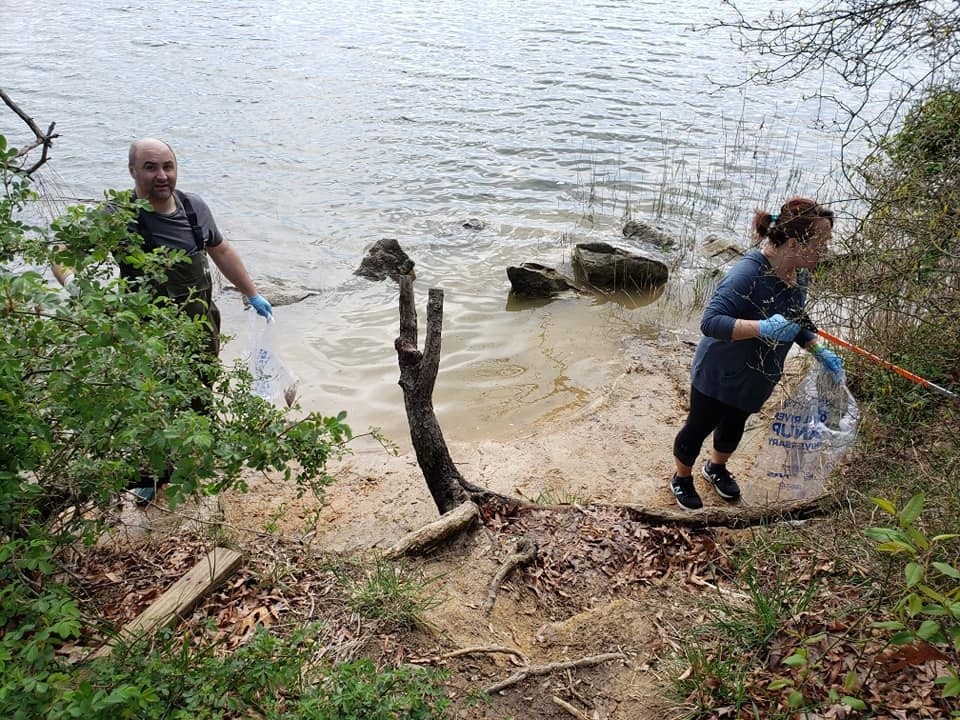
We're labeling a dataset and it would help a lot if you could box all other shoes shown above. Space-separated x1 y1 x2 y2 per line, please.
135 487 156 506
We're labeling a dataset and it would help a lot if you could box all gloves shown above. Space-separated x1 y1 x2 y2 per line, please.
64 274 93 299
760 314 800 342
248 293 272 319
816 347 841 375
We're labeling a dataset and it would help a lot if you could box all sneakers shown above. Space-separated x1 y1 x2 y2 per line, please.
702 459 740 498
670 472 704 515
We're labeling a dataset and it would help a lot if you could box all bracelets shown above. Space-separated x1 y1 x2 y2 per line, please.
808 342 822 352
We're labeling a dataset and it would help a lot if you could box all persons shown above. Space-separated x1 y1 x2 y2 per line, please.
669 199 842 509
52 138 272 421
